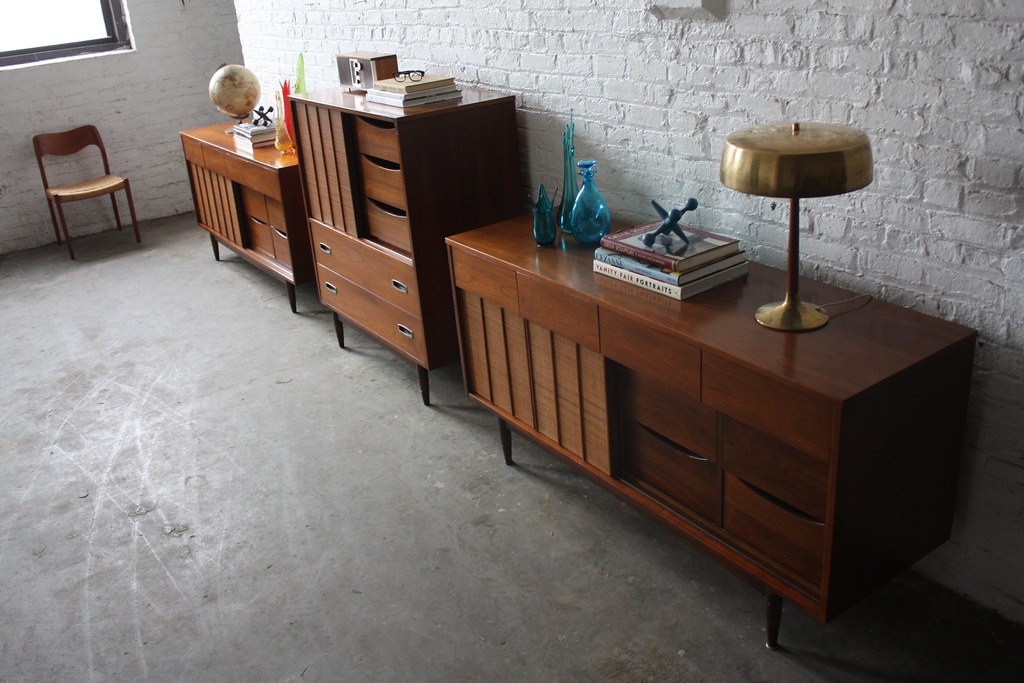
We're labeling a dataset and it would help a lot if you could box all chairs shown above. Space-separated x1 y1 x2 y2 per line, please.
32 125 141 260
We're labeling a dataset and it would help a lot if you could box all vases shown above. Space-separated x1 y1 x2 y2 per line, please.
294 53 306 95
272 90 296 156
558 108 580 233
279 78 297 149
528 184 560 245
568 159 611 244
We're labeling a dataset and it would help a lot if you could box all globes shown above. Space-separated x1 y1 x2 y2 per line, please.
209 63 261 134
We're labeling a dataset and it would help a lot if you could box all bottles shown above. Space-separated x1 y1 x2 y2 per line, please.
571 159 612 246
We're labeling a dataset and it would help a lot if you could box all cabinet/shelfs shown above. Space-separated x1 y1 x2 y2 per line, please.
444 209 979 649
179 119 316 313
287 82 525 407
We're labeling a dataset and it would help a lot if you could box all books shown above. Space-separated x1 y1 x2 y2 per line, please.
601 221 741 270
592 245 748 300
365 73 465 107
233 117 277 149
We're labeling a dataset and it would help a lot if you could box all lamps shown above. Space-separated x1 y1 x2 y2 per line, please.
720 120 874 332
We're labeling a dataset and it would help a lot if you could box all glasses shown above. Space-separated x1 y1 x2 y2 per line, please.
392 70 425 83
